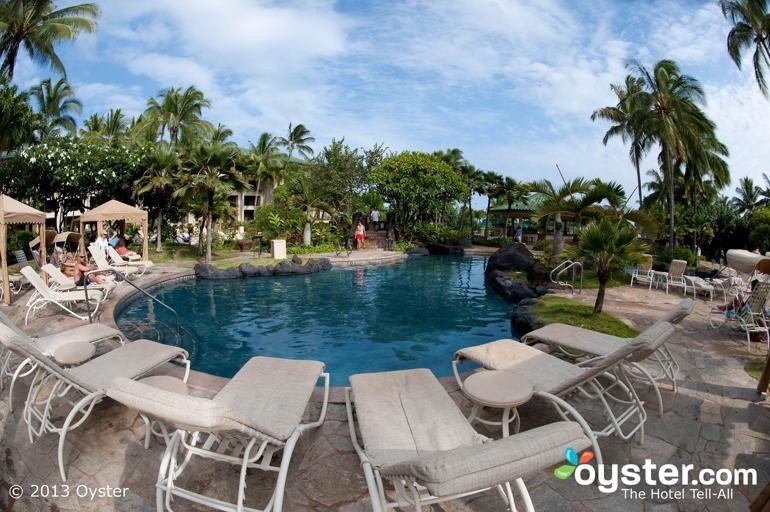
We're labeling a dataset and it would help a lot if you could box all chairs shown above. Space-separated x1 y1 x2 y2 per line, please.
628 253 770 352
0 298 697 512
1 242 155 328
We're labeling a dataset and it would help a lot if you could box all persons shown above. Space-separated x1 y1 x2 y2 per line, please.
715 278 761 319
25 221 129 291
354 219 367 249
494 222 522 245
351 267 364 297
371 207 380 233
133 222 208 244
